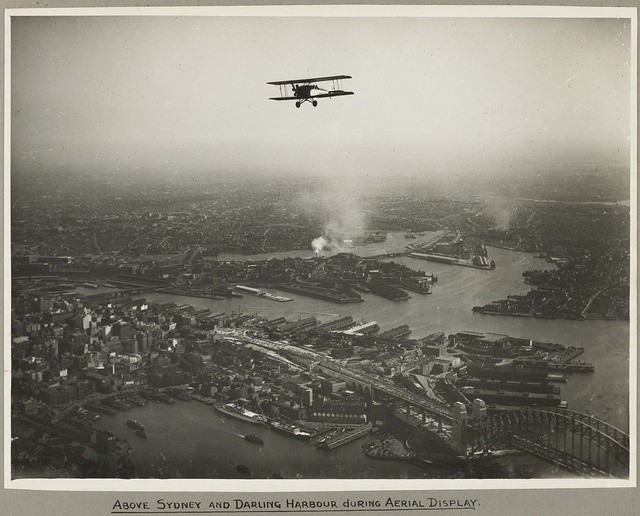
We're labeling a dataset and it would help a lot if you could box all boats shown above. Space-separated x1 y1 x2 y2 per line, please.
126 417 145 430
244 433 263 445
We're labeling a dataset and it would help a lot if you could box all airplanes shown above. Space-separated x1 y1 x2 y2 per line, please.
267 75 354 107
